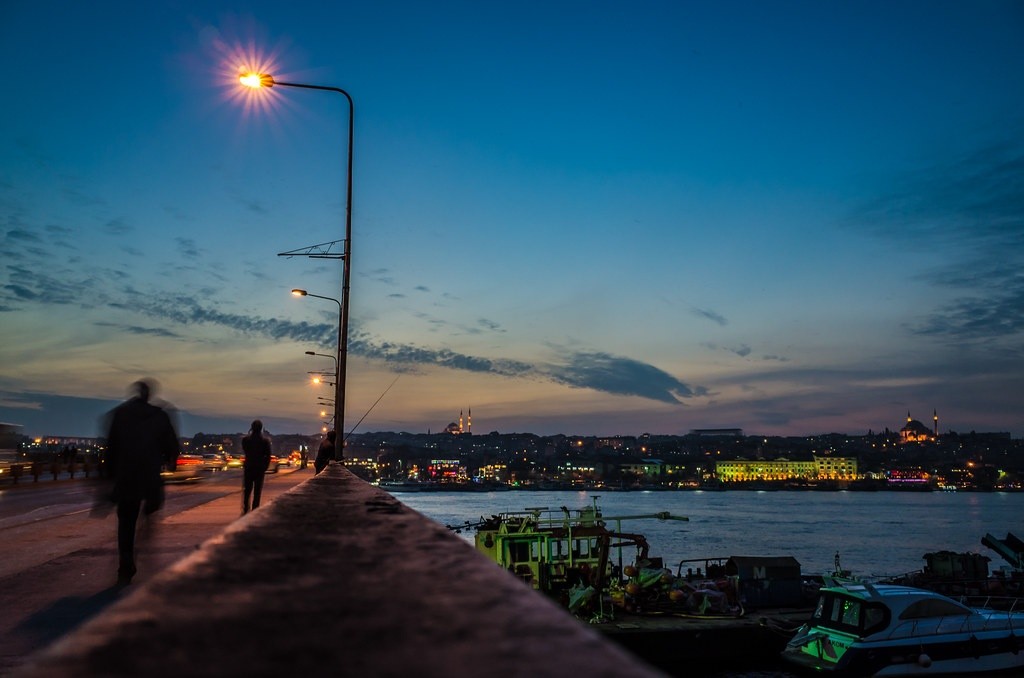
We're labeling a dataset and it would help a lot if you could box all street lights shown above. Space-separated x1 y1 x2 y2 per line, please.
239 70 355 465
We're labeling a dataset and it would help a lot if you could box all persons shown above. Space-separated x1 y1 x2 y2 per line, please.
314 431 336 475
242 420 272 514
92 378 179 587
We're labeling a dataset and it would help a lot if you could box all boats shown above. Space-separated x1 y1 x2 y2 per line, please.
441 495 1024 678
378 481 616 492
